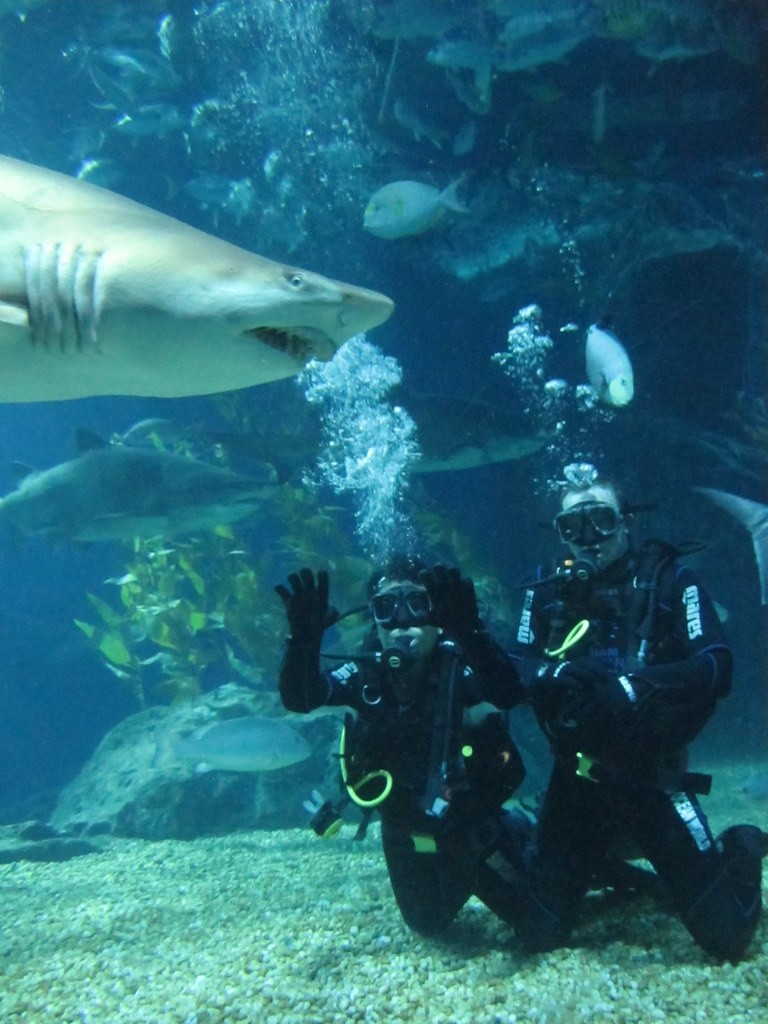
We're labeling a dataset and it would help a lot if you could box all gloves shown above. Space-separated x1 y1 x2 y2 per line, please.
536 656 599 691
596 667 661 729
275 569 337 637
417 562 483 640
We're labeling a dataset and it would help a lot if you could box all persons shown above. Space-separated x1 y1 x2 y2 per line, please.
272 462 768 963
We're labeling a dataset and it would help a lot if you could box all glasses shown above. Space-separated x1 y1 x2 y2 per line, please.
555 506 619 542
365 588 433 624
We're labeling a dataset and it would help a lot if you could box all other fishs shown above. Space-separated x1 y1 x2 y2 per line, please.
112 418 175 447
79 0 723 286
586 324 635 408
155 718 312 775
